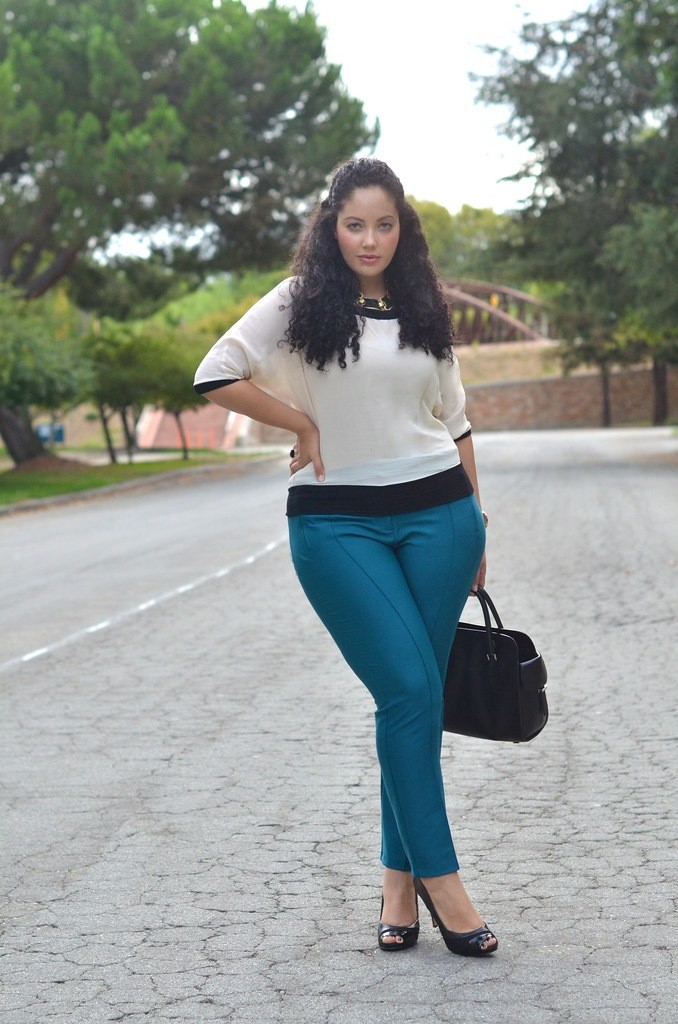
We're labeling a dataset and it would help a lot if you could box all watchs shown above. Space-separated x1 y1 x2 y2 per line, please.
482 511 488 527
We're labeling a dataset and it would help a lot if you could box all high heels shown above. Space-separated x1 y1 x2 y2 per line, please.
413 876 498 955
377 890 419 950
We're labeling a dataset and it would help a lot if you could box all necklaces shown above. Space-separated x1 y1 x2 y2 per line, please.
356 291 393 311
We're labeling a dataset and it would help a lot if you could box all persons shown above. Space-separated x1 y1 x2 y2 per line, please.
192 160 497 955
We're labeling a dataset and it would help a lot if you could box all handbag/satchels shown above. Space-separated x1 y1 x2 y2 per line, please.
441 587 549 744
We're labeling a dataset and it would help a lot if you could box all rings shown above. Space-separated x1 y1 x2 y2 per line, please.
290 449 295 457
